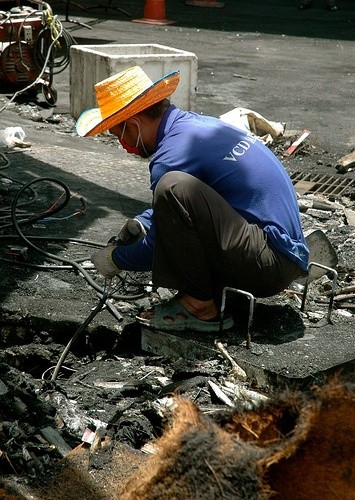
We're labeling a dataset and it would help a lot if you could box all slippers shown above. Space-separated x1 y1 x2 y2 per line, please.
136 297 234 330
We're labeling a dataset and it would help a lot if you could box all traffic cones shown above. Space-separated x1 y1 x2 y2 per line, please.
131 0 176 25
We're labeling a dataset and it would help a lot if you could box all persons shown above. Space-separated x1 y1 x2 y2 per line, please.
74 65 311 334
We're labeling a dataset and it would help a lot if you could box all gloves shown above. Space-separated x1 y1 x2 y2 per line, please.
91 218 147 279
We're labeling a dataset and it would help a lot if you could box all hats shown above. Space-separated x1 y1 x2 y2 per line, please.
75 64 180 138
